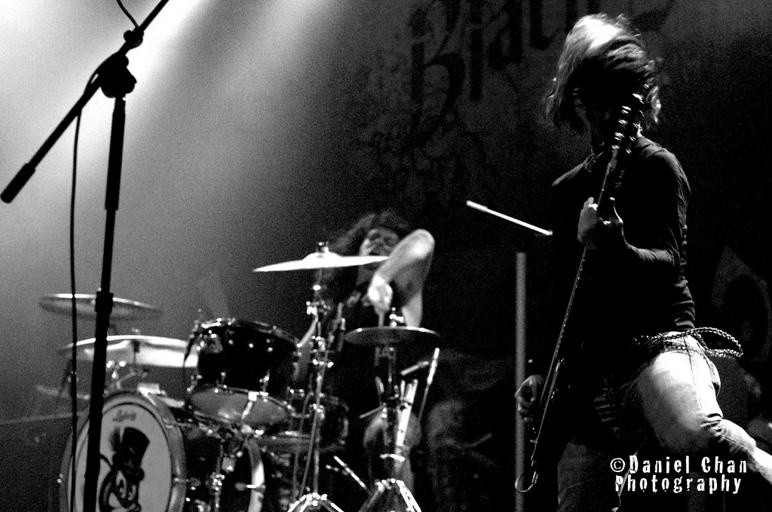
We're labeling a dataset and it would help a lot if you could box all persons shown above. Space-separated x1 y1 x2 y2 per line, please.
296 207 436 512
514 13 772 512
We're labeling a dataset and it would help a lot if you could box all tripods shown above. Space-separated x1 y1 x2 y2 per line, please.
352 401 425 512
281 399 345 512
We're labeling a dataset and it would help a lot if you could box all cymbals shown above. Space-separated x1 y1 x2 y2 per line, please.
252 252 391 273
58 335 200 368
39 294 162 321
345 327 441 347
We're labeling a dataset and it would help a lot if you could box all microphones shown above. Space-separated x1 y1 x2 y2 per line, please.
464 195 556 244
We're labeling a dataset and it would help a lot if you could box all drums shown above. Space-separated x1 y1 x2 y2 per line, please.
188 320 301 425
265 388 347 457
57 389 265 512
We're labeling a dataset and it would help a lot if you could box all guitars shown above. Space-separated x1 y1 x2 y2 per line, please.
523 85 652 473
